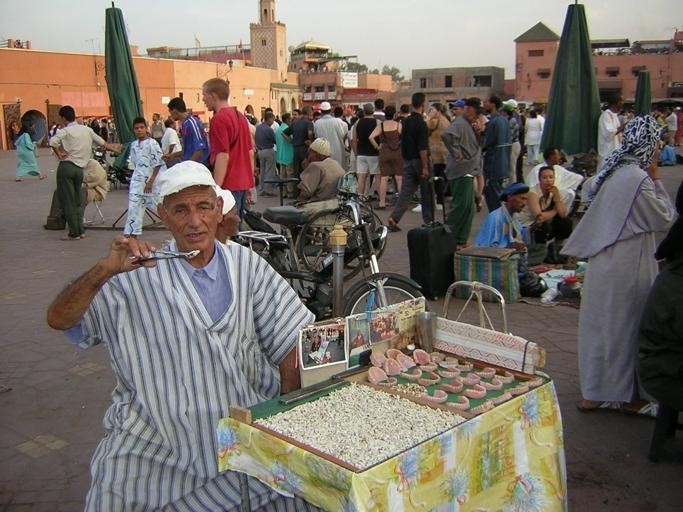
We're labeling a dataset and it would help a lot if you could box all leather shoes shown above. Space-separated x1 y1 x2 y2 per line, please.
368 195 377 203
373 203 386 211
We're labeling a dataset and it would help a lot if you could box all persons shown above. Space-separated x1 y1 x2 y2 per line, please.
246 91 586 276
557 112 680 420
368 309 402 345
635 180 681 465
50 97 211 239
45 159 317 511
300 326 344 368
201 78 256 230
596 92 683 173
353 329 364 347
10 116 48 183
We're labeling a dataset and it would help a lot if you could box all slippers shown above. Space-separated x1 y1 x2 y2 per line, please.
576 396 660 418
386 224 401 232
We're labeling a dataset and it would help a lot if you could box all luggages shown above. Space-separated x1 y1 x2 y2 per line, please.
406 221 459 302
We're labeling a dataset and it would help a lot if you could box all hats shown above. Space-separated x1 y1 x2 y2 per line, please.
151 159 236 215
449 99 465 108
500 183 529 203
309 137 331 157
365 103 373 111
502 98 518 108
320 101 331 110
502 104 514 111
673 106 681 111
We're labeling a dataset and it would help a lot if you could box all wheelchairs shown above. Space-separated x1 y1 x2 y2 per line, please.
292 169 389 284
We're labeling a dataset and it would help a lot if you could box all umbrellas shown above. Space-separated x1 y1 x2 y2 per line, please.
539 0 601 165
101 2 146 177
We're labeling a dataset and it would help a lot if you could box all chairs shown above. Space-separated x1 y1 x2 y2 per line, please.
94 182 111 223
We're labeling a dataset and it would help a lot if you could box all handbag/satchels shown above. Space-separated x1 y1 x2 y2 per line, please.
337 171 359 196
526 244 548 266
519 271 549 299
543 241 569 265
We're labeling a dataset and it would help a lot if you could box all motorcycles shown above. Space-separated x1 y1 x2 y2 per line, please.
228 194 430 346
93 144 132 188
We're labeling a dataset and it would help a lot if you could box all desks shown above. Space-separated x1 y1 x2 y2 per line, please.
217 349 569 512
263 177 299 206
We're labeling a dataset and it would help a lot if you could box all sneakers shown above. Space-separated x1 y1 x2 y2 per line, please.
412 204 422 213
